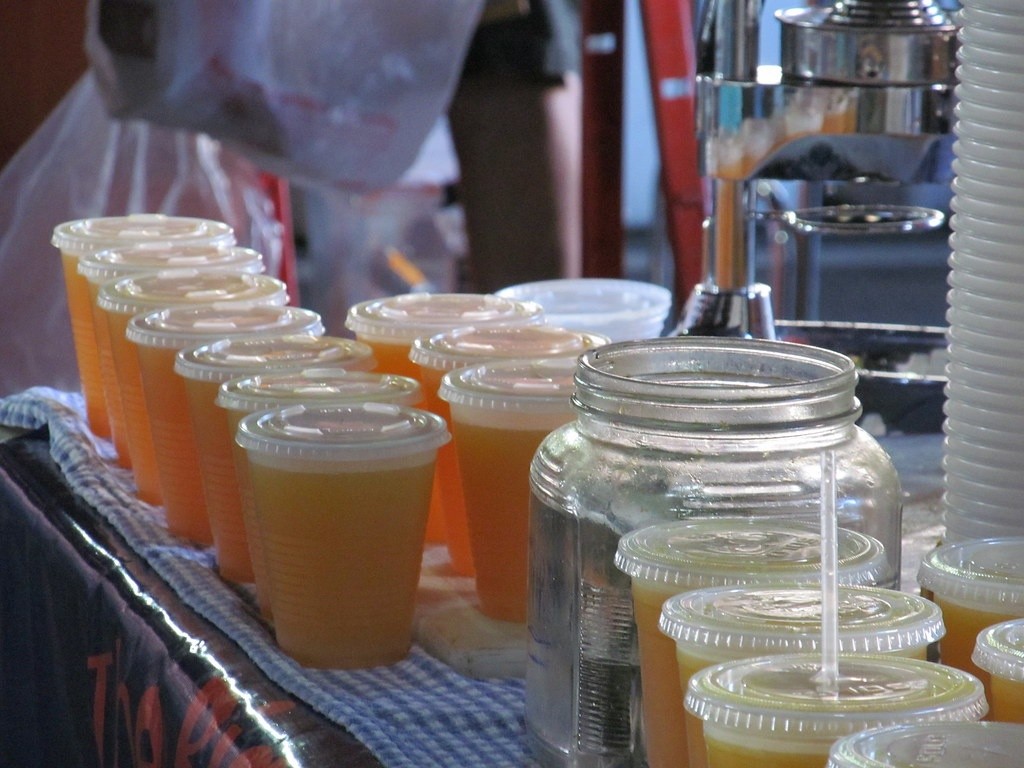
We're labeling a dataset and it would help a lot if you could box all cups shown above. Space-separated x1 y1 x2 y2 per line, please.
497 279 672 344
52 209 450 666
614 521 1023 766
437 362 580 625
343 292 544 541
411 328 612 576
940 2 1024 544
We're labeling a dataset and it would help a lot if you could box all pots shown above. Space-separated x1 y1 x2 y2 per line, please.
774 7 959 87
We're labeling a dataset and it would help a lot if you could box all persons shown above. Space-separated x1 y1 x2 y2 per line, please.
0 0 582 291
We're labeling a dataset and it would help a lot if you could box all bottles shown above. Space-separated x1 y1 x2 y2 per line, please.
530 335 900 766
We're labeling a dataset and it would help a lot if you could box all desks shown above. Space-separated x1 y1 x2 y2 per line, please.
0 385 561 768
